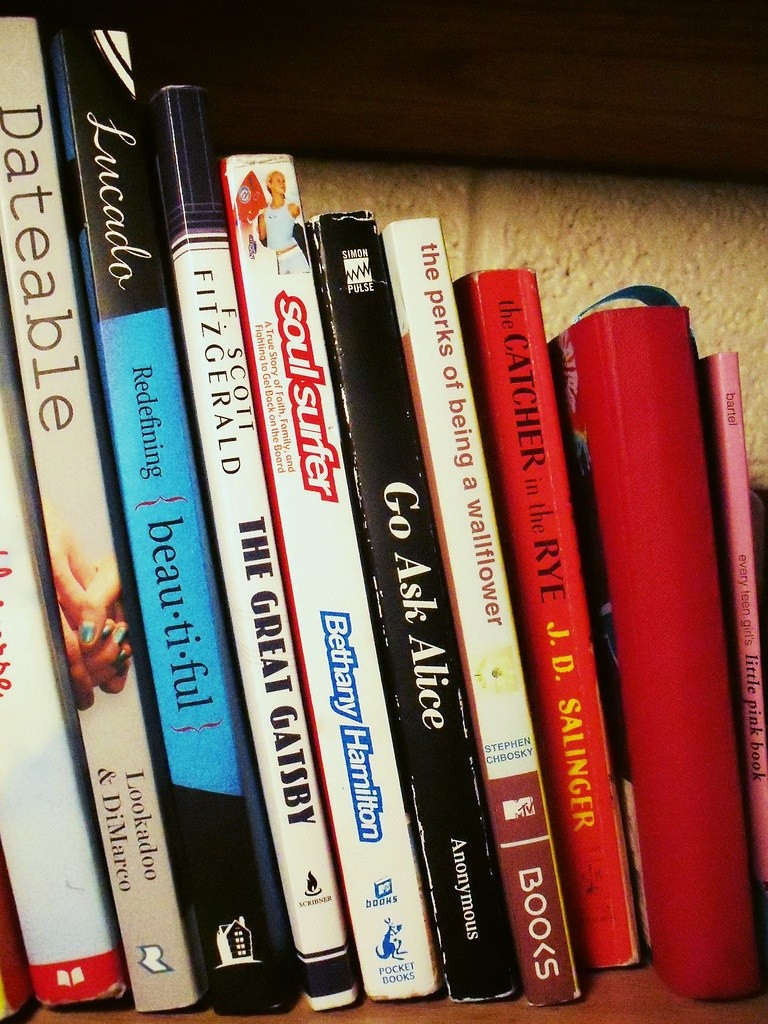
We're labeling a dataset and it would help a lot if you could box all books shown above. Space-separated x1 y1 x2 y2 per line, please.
0 7 768 1024
